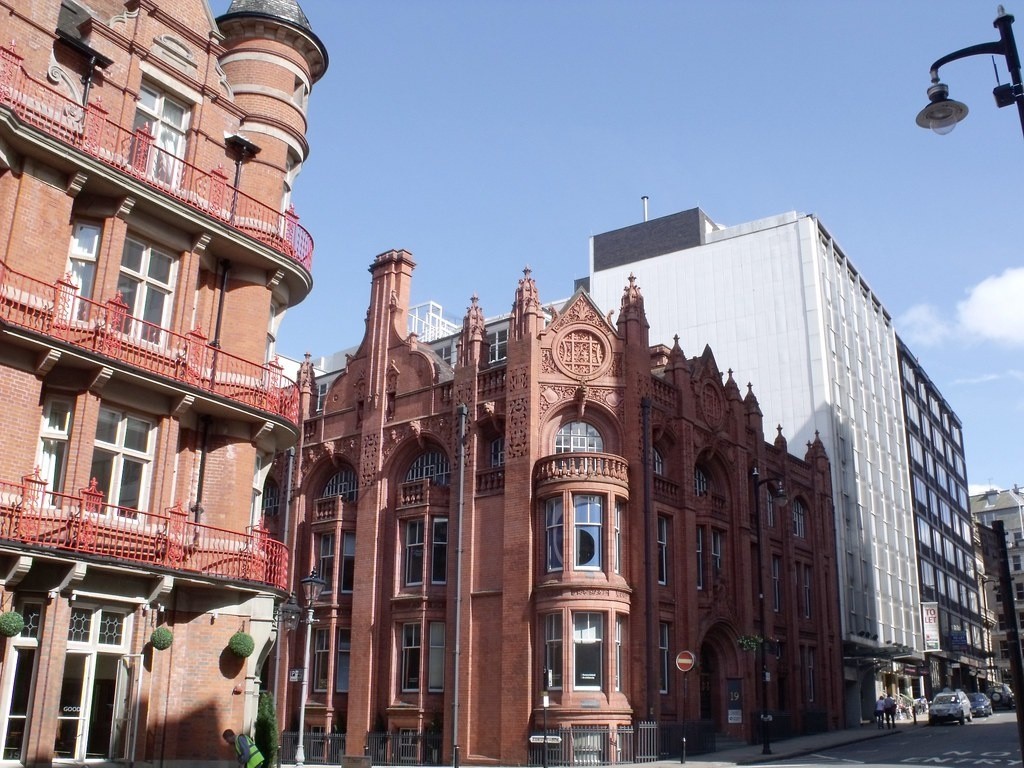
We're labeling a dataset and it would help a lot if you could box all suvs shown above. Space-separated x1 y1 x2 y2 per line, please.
986 683 1016 709
929 691 972 726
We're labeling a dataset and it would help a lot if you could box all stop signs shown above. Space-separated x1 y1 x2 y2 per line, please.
675 651 695 672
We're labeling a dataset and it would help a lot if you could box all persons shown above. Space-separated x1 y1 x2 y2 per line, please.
884 695 896 729
920 696 927 714
222 728 265 768
873 696 886 730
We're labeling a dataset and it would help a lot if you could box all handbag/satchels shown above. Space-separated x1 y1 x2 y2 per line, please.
874 711 878 716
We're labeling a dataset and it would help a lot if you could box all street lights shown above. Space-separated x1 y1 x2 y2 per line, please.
981 578 999 687
753 468 789 753
915 5 1024 141
295 566 327 768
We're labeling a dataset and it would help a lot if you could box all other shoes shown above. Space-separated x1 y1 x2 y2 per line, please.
882 725 884 729
888 725 890 729
893 725 896 728
878 726 880 729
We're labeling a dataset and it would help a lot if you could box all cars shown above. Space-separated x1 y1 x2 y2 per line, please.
966 693 993 718
